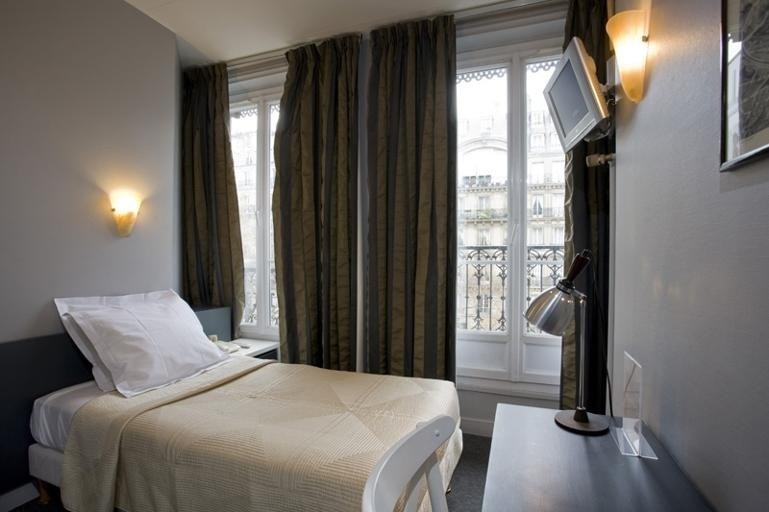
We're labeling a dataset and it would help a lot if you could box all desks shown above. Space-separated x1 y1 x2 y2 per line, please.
480 400 717 512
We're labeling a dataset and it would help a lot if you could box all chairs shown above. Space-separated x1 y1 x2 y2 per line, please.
361 415 457 512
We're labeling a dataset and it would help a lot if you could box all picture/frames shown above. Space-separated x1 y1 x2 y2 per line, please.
720 1 769 175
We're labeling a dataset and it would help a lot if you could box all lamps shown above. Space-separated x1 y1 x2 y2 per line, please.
604 7 654 104
524 247 609 436
108 185 144 237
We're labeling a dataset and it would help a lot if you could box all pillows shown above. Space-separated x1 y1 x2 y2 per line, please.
54 285 235 396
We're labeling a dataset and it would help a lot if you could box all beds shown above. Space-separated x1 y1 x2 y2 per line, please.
24 334 465 512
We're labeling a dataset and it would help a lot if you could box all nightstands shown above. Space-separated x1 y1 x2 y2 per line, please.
215 336 282 363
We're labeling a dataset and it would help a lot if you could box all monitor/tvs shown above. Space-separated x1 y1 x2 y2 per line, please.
543 36 610 155
